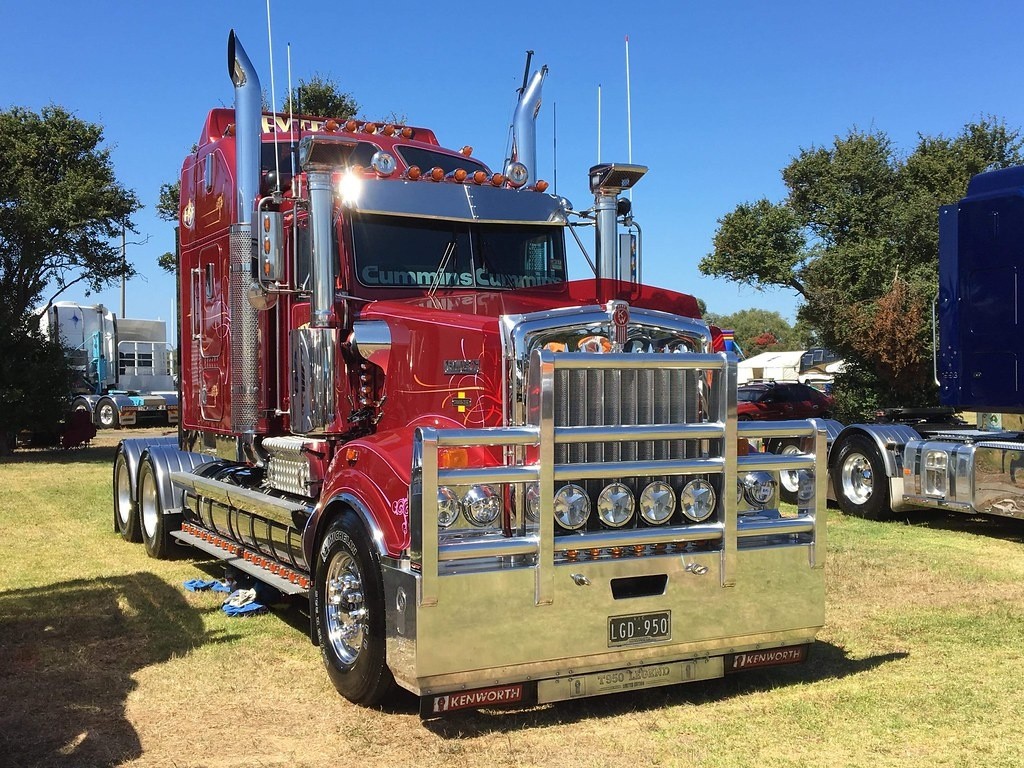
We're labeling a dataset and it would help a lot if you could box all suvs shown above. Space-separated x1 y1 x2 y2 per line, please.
736 378 832 423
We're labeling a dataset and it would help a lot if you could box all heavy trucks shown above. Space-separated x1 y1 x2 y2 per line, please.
30 302 180 427
112 25 832 715
766 166 1024 524
